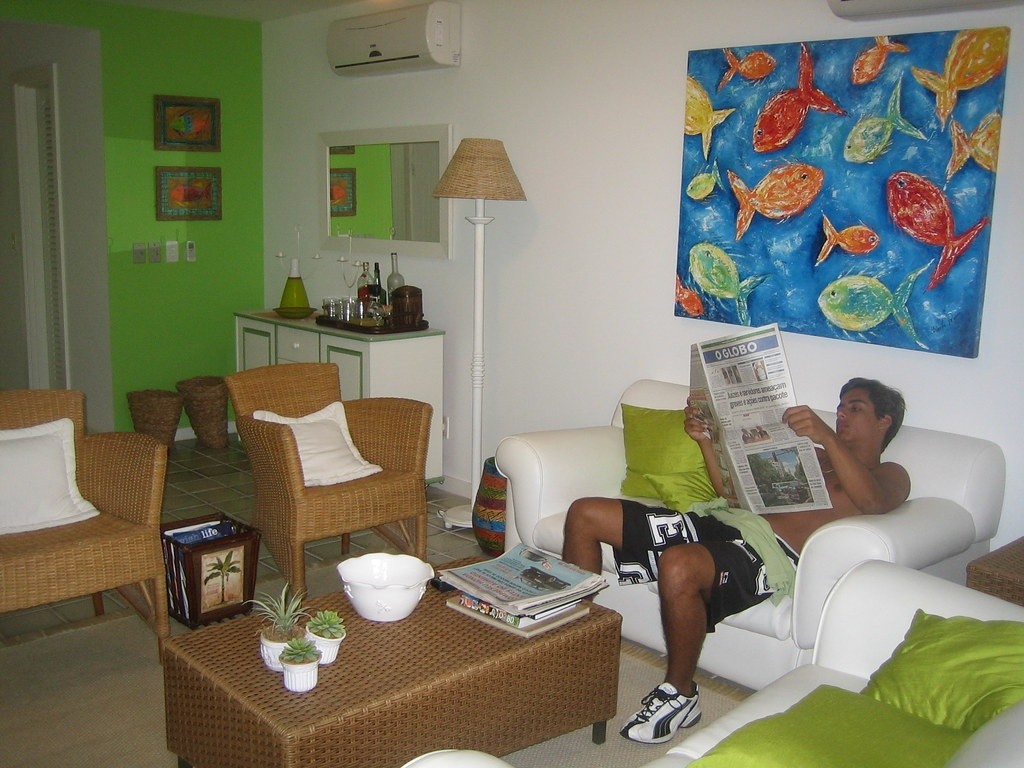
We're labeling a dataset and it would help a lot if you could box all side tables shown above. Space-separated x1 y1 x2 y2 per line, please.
965 537 1024 607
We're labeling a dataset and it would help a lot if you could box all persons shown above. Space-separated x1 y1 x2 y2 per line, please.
562 377 912 744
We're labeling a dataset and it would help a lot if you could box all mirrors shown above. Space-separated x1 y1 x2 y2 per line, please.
318 122 453 260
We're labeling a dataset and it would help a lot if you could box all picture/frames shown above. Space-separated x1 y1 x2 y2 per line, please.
153 94 222 153
156 165 223 221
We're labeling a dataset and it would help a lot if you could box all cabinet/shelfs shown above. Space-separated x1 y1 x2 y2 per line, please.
233 310 445 490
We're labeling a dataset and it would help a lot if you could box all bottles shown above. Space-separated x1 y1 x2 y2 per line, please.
280 259 309 307
387 253 404 316
358 262 386 319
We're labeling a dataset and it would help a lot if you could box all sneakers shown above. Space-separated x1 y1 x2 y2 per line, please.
619 680 701 743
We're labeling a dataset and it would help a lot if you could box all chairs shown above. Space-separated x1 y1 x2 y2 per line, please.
224 362 433 600
0 388 170 638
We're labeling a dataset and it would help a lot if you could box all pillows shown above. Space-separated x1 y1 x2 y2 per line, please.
859 606 1024 732
641 469 720 513
685 684 971 768
252 401 384 489
0 418 101 535
619 402 705 499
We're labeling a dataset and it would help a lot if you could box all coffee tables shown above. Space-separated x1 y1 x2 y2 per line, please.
161 555 622 768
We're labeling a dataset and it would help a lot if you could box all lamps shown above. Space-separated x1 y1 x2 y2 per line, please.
432 139 527 530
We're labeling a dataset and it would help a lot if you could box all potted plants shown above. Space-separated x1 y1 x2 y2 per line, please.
304 609 347 664
279 639 323 692
241 580 318 672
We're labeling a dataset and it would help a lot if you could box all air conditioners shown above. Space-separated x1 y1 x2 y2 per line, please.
326 1 461 76
827 0 999 17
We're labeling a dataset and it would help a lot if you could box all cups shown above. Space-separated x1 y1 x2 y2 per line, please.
323 298 363 322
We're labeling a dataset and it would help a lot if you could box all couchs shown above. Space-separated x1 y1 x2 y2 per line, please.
401 558 1024 768
495 378 1008 691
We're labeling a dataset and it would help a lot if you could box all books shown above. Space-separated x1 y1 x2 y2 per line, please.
161 517 238 617
445 592 590 638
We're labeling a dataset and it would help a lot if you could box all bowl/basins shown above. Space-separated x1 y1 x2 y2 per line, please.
273 307 317 319
337 552 434 622
